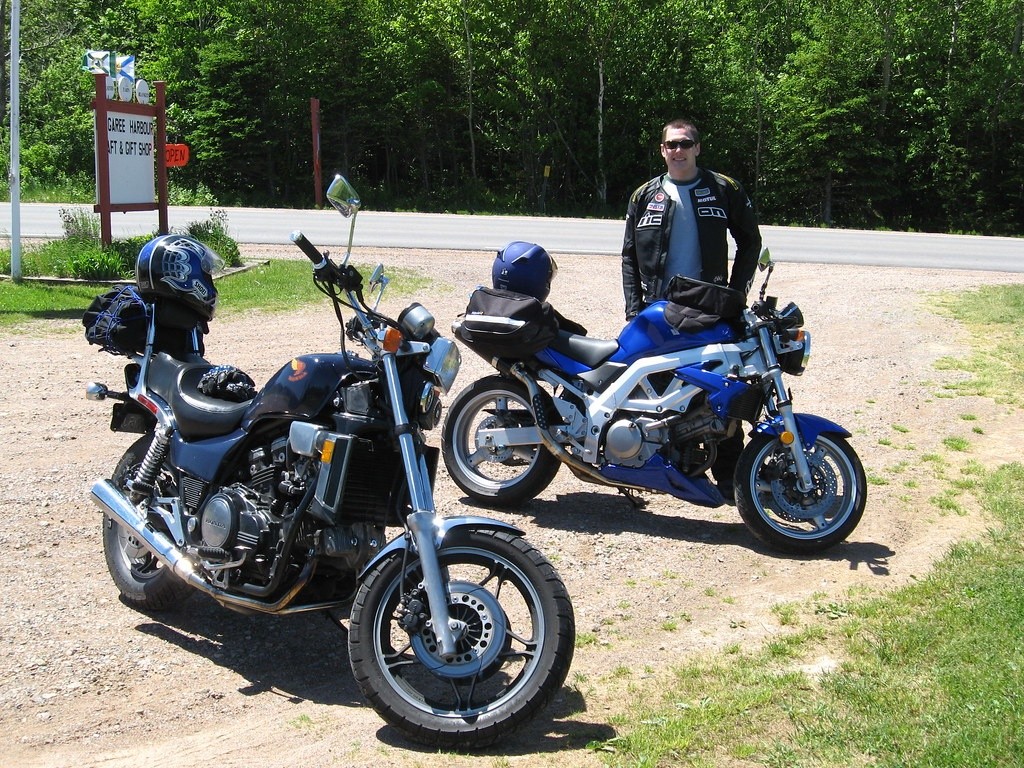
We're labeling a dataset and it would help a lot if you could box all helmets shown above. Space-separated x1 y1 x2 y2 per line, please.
491 242 551 303
134 234 219 321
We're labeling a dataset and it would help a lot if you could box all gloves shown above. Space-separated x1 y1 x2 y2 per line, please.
197 364 258 402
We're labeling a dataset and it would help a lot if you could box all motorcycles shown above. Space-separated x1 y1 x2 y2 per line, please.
439 238 868 557
80 174 575 751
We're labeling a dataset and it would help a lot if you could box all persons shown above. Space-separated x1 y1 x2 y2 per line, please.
622 118 762 506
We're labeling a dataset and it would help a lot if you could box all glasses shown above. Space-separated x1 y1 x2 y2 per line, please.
662 139 697 150
